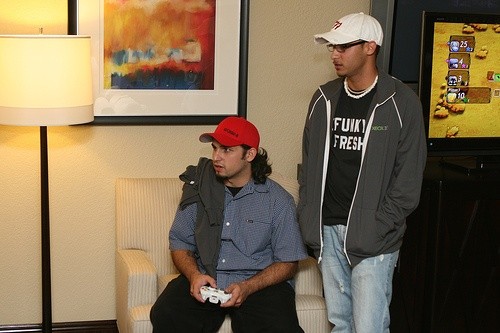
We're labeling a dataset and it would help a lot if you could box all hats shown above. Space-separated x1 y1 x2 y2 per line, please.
199 116 260 152
314 12 384 46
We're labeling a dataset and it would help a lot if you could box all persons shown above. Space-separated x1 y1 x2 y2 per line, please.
295 12 426 333
150 115 306 333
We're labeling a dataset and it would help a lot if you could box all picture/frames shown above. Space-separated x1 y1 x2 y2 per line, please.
68 0 250 125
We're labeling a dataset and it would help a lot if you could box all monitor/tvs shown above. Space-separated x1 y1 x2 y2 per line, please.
418 10 500 150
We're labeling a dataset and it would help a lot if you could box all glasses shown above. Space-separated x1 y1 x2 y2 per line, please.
327 40 367 53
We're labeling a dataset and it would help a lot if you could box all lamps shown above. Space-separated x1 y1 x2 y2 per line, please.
0 35 94 333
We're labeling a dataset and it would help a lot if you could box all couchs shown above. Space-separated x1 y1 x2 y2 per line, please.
114 177 332 333
